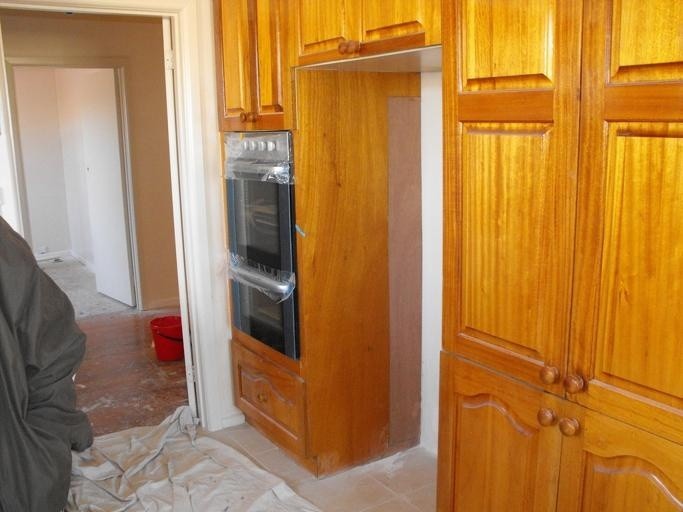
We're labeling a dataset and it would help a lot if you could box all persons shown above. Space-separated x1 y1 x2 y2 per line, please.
0 216 96 511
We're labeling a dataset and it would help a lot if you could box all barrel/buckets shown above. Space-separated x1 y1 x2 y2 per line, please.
149 315 183 362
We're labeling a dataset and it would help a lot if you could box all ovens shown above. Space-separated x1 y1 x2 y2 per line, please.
224 131 298 356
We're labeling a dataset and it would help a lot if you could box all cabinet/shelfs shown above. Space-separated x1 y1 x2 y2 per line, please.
435 1 683 512
211 0 443 479
